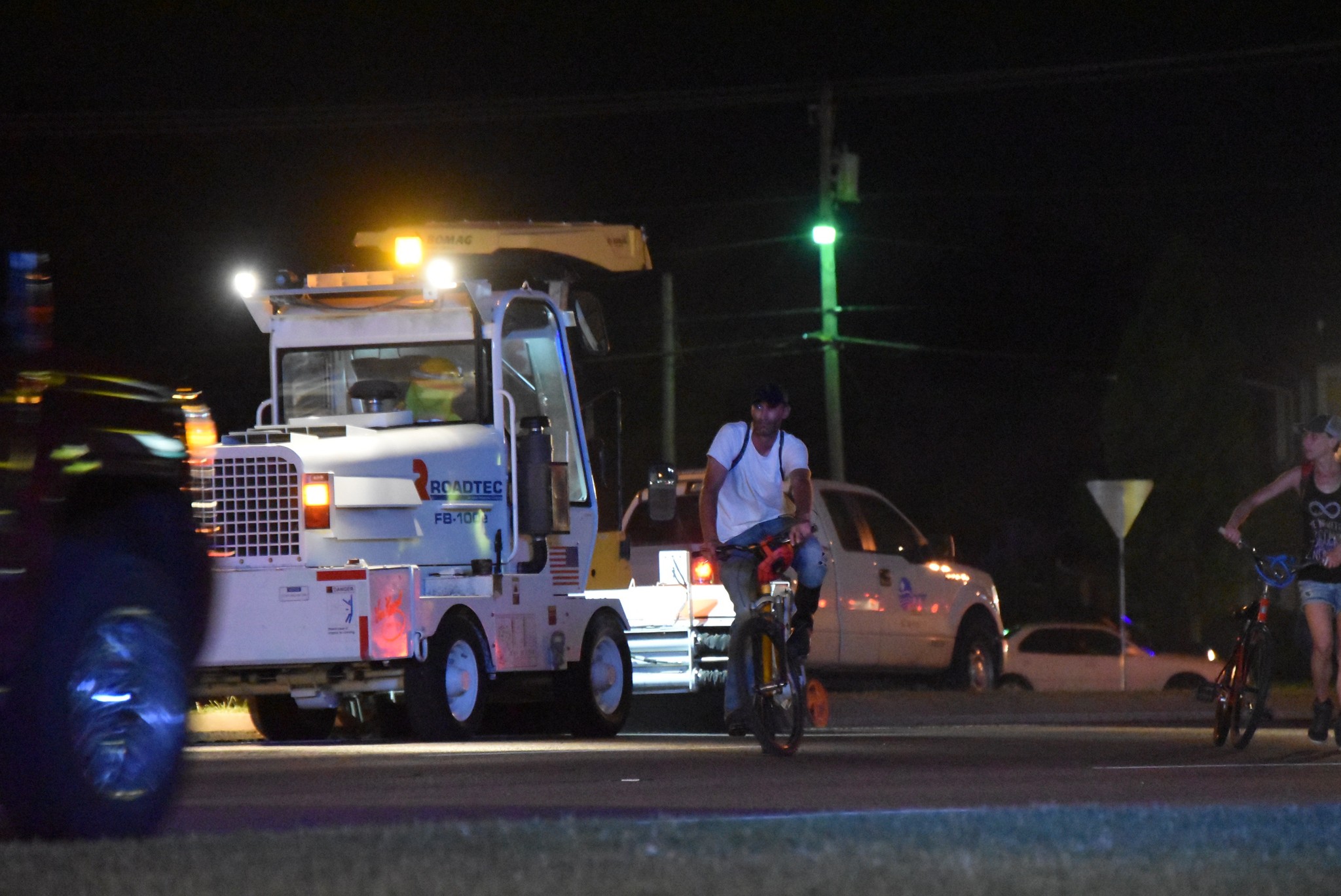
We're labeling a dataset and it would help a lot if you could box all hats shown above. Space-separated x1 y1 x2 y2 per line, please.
748 383 789 405
1298 414 1341 442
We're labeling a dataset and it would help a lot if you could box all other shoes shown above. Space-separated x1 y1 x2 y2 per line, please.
729 705 749 735
787 630 810 661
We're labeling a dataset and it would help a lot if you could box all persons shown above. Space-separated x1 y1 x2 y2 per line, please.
698 386 828 737
1224 416 1341 748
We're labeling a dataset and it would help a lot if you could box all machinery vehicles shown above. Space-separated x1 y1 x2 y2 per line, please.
189 219 791 745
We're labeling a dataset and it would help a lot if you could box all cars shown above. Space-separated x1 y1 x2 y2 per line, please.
0 366 222 841
620 468 1005 692
999 623 1248 691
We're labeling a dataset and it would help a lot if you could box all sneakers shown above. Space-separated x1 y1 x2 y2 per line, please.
1334 711 1341 747
1308 696 1332 740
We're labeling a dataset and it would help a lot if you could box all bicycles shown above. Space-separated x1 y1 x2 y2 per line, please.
690 523 822 764
1196 526 1322 751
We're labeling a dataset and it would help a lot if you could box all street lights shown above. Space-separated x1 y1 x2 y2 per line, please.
812 214 843 481
